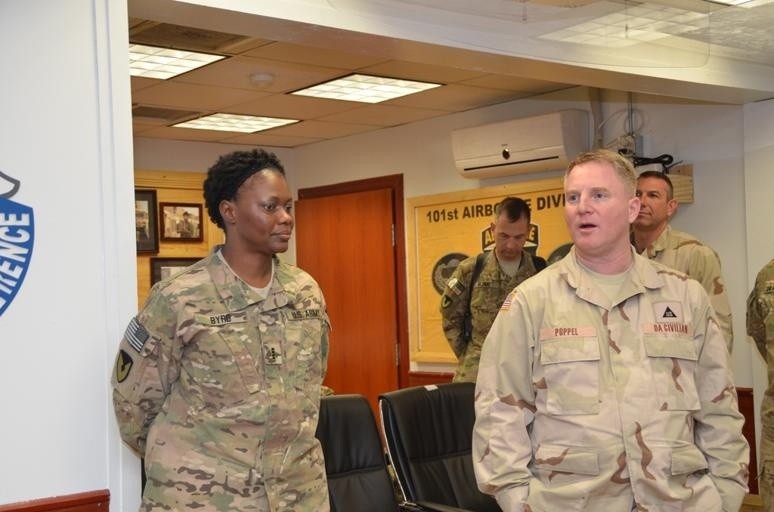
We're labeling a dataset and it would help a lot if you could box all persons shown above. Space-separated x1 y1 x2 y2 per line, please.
138 221 149 241
632 169 735 357
745 259 773 510
111 146 334 512
437 197 549 385
471 150 750 512
176 210 194 237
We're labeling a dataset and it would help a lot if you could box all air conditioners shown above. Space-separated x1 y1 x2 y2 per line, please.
449 109 589 182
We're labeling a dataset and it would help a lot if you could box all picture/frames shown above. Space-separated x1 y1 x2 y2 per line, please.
134 186 205 286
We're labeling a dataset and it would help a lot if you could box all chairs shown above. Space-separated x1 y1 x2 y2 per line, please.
314 382 503 512
